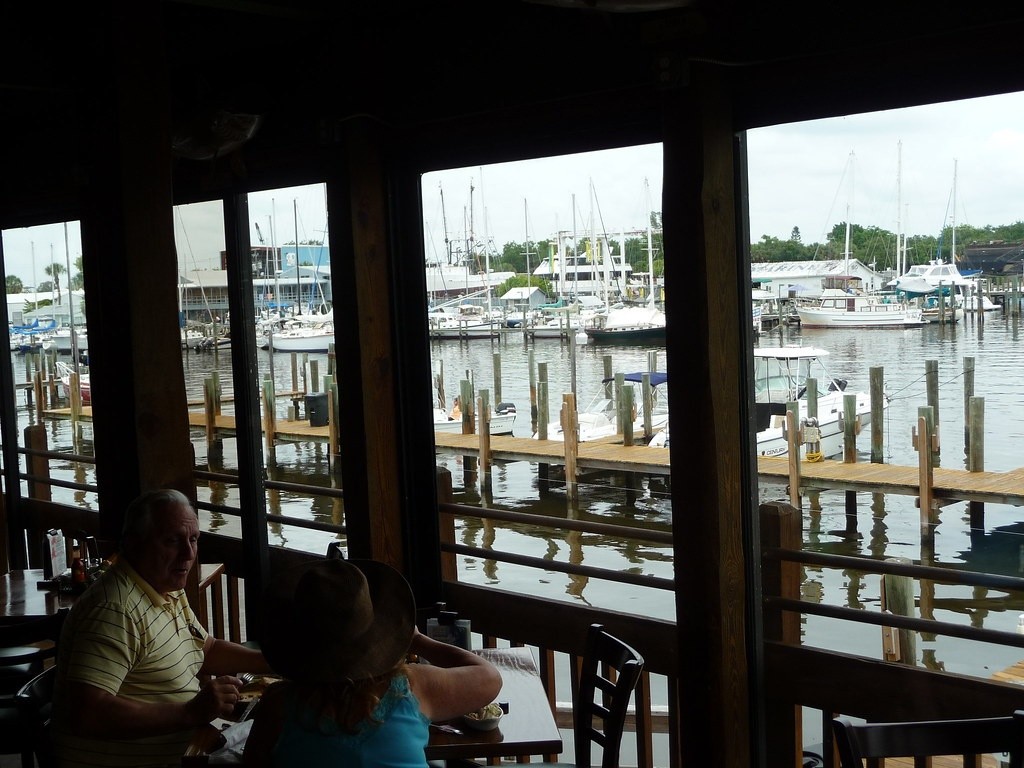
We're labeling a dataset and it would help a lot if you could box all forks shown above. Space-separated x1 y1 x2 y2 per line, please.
237 673 253 689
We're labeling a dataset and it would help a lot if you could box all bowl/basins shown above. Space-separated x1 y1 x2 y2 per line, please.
463 706 503 731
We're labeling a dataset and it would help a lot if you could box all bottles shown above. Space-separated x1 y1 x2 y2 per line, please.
70 545 84 581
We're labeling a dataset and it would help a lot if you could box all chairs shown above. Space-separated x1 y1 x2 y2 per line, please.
0 609 71 768
833 709 1024 768
480 623 646 768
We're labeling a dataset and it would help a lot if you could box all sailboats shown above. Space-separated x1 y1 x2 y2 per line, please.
10 138 1003 359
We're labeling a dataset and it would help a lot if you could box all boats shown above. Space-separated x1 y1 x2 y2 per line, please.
648 346 894 461
53 361 90 404
532 370 668 442
433 402 517 436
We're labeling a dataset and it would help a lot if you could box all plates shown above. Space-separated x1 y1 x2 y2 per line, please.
236 674 283 702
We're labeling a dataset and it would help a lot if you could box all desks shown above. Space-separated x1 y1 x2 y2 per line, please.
184 646 564 768
0 563 224 649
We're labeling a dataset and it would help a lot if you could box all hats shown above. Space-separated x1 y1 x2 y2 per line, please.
283 560 416 681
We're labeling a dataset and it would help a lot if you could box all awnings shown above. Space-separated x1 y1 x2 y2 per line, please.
897 278 935 293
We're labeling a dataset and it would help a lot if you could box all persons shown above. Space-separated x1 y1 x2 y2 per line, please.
241 559 502 768
47 488 272 768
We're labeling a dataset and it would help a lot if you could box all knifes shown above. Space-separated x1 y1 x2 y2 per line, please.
239 697 258 722
